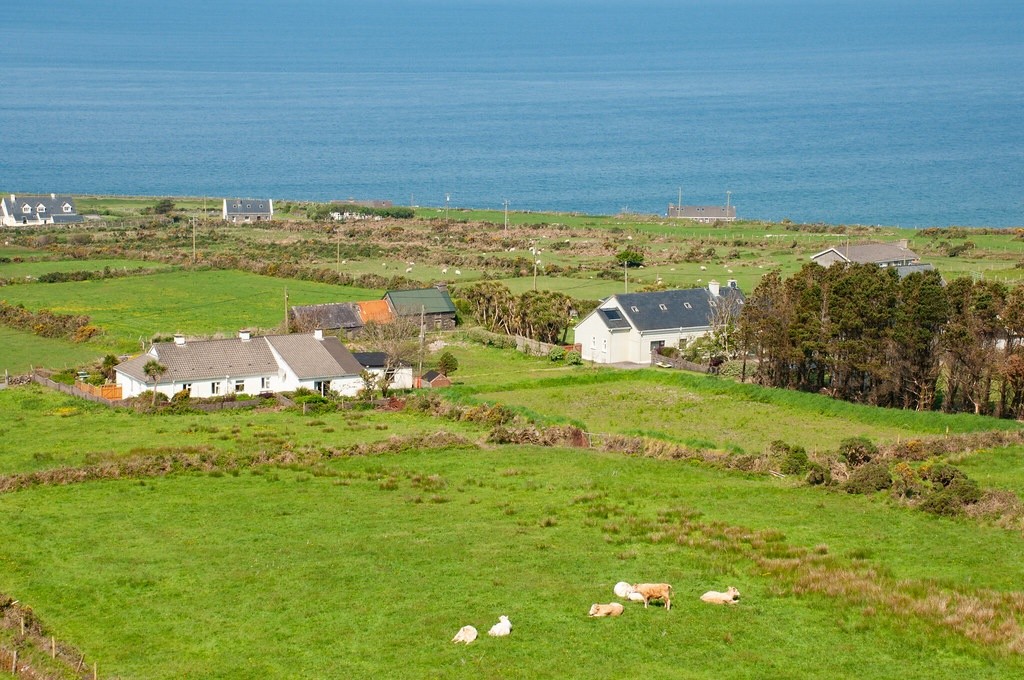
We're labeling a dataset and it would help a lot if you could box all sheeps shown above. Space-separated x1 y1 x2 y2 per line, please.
613 581 675 611
337 228 768 290
488 615 512 637
452 625 477 645
589 602 624 617
700 586 741 605
